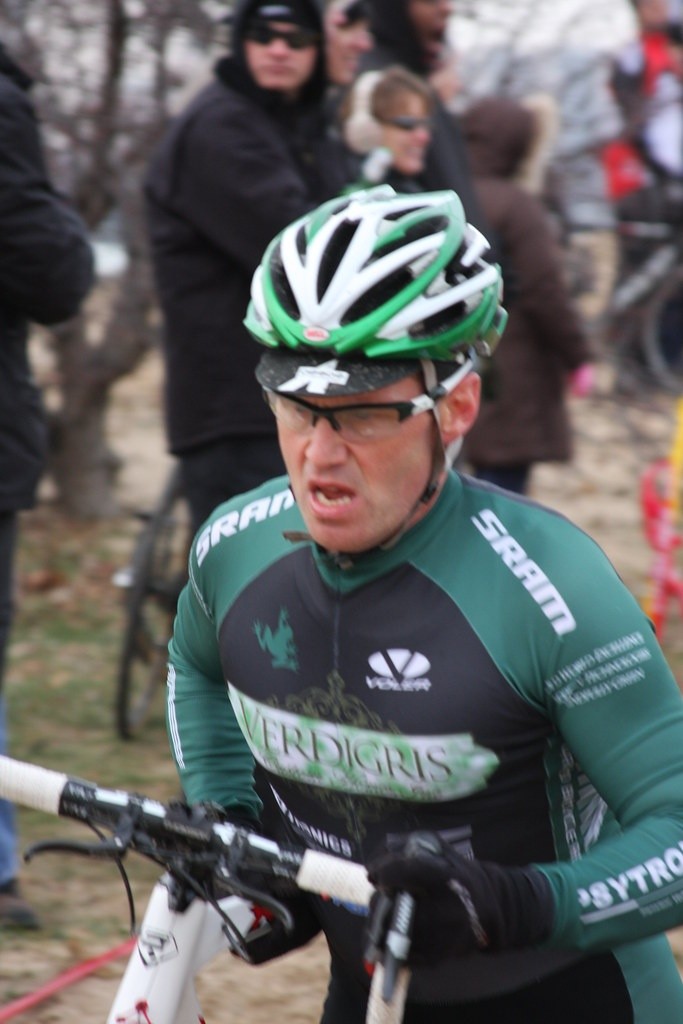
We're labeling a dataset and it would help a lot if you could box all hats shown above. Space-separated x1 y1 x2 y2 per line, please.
252 349 421 394
230 0 326 32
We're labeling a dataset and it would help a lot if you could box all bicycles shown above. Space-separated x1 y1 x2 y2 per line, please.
114 463 199 738
0 750 477 1024
546 150 683 384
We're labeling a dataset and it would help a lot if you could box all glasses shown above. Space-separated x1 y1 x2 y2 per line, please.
383 115 431 131
246 21 315 51
264 358 474 442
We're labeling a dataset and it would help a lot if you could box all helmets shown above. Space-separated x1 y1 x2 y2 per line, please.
242 182 510 370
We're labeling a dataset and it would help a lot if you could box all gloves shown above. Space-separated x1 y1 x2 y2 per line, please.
367 832 555 970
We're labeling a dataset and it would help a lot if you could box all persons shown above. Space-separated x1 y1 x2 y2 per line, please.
0 50 96 927
590 0 683 406
166 186 683 1024
145 0 592 534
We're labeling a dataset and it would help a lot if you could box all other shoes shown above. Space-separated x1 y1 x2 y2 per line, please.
0 887 38 929
